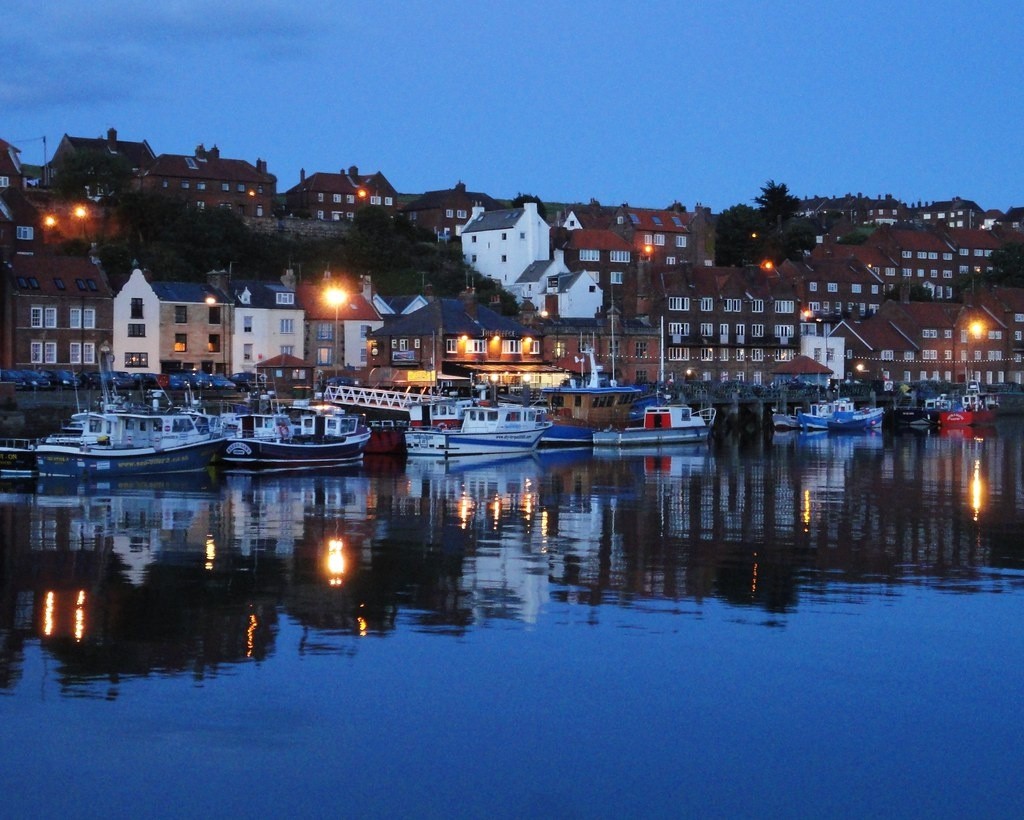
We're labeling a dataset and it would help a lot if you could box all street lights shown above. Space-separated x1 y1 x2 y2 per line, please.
325 288 346 378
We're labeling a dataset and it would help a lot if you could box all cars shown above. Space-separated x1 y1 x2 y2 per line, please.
206 373 237 391
0 367 190 391
228 372 263 392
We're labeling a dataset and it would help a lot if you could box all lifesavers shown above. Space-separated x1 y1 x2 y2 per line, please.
437 422 448 430
279 425 290 437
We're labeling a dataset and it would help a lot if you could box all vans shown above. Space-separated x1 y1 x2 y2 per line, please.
176 368 214 389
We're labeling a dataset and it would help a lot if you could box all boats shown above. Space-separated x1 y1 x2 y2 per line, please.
942 426 998 439
590 441 714 540
798 428 884 504
770 381 1001 428
590 403 717 447
771 430 799 448
321 380 477 457
398 400 553 457
33 476 229 590
402 452 534 552
221 457 368 559
217 365 372 464
31 345 228 480
525 280 669 447
